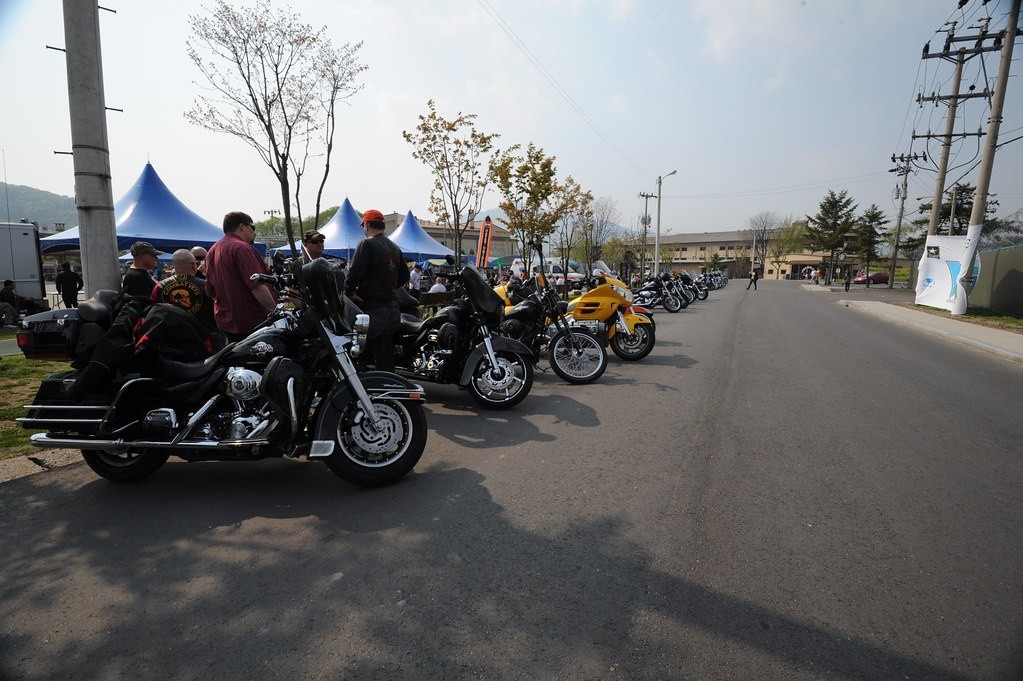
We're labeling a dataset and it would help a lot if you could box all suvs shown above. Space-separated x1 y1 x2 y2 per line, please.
530 265 585 282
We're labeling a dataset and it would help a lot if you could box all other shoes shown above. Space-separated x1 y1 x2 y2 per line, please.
4 322 19 328
746 288 749 290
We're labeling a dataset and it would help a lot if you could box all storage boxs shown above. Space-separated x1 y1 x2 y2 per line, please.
22 370 77 431
17 308 84 362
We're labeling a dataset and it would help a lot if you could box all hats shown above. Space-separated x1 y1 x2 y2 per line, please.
415 265 422 271
131 241 163 257
360 209 385 226
303 230 325 242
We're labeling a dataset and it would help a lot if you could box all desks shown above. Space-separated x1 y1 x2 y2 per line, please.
46 291 85 310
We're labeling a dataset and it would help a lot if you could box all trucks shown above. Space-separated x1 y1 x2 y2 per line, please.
510 257 581 279
0 220 51 318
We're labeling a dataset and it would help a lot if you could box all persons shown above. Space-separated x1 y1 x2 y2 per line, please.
0 210 564 363
808 267 864 293
745 268 758 290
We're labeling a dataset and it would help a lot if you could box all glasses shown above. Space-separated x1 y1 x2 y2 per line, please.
307 239 325 244
195 256 206 260
245 224 255 231
194 262 199 267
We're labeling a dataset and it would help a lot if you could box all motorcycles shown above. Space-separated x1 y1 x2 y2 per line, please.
395 255 534 409
14 248 428 485
494 268 730 385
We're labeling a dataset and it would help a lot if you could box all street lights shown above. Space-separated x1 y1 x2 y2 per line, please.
655 169 677 277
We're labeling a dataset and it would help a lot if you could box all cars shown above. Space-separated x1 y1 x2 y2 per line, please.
853 272 889 284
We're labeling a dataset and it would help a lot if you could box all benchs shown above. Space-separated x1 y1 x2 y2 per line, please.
419 290 456 317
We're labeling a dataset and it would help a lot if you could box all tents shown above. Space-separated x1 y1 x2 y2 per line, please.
39 163 463 267
